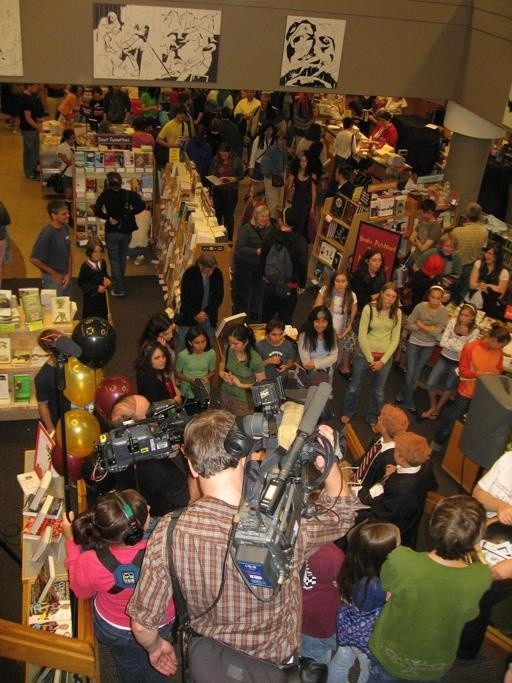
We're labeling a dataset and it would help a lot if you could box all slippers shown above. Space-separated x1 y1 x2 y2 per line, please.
428 411 440 419
339 368 353 382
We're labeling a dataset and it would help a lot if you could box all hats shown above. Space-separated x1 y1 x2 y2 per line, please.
309 546 345 581
422 255 445 276
282 206 297 229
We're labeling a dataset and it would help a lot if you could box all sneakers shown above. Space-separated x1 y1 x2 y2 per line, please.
394 396 419 415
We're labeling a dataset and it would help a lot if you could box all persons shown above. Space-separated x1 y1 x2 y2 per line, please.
0 82 512 683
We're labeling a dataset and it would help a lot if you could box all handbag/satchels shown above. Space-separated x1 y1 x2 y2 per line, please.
116 209 138 235
342 326 357 352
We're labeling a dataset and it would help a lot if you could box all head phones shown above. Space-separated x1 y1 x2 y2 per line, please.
109 488 143 546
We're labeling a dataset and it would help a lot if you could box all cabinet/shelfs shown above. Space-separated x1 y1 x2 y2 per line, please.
0 117 512 683
